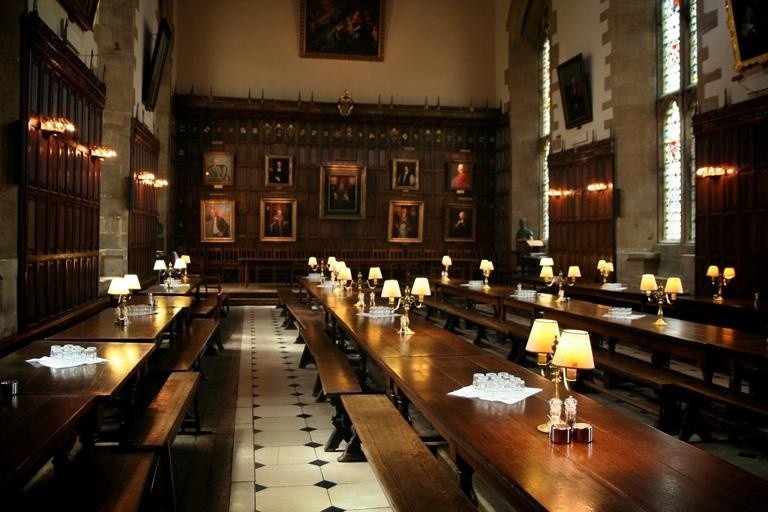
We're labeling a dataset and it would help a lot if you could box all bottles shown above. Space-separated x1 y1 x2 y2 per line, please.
147 289 152 307
564 396 577 426
154 299 158 306
549 398 563 433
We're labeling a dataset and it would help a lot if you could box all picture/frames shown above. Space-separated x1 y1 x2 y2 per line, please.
298 0 386 63
58 0 98 31
142 18 171 111
555 51 593 129
723 0 768 73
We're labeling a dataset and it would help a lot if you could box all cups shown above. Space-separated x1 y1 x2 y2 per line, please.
514 289 537 298
47 343 97 363
163 280 181 287
116 304 152 316
369 305 395 317
472 371 526 396
608 306 632 317
572 423 592 443
550 425 572 444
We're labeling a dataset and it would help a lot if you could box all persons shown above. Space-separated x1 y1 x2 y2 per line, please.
451 163 469 188
516 217 533 240
210 207 229 237
395 208 414 238
274 161 284 183
397 166 415 186
207 158 224 181
329 10 377 56
454 212 469 238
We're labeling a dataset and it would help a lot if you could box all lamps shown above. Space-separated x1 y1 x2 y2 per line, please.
444 203 476 243
200 199 235 243
388 199 424 244
202 152 234 188
319 165 367 221
265 154 294 189
391 158 420 192
259 197 298 243
447 162 475 194
